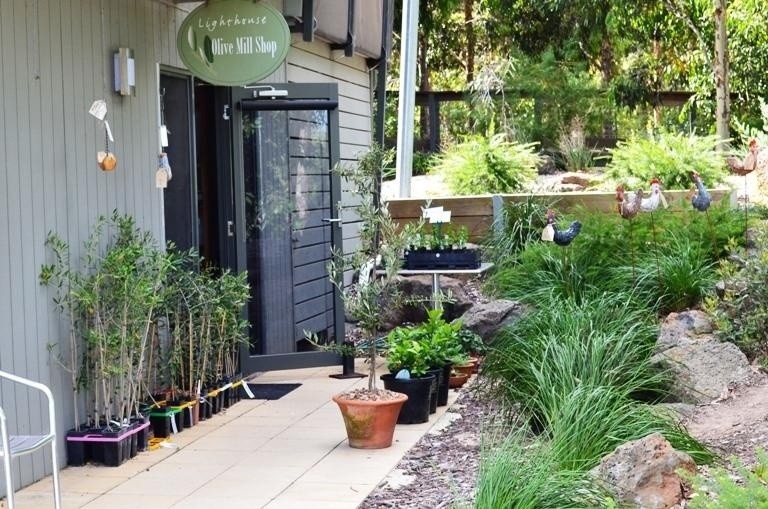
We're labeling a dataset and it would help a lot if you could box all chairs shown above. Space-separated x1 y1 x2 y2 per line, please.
0 370 61 509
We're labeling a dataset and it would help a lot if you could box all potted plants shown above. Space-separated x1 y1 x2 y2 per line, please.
303 137 483 448
37 208 255 466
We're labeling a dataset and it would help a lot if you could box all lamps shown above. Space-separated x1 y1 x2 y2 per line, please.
114 47 136 97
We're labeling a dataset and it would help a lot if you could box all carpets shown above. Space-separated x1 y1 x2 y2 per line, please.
242 383 303 400
